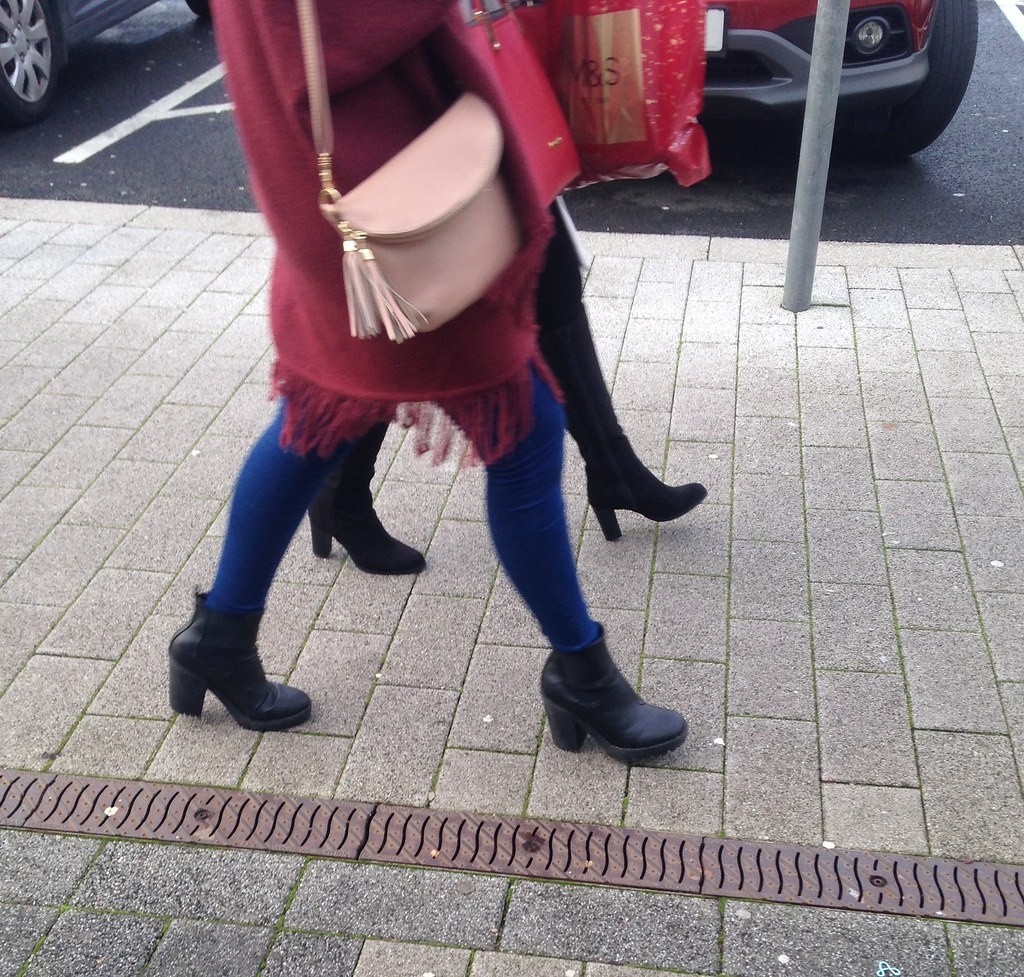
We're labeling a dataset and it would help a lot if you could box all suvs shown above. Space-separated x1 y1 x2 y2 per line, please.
692 0 977 157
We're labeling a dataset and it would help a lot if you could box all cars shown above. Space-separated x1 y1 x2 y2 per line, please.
0 0 213 130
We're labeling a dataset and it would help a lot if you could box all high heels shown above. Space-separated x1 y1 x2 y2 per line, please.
168 593 312 730
542 622 689 760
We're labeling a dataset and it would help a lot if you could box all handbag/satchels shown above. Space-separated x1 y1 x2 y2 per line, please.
555 0 712 192
319 93 519 342
444 3 581 208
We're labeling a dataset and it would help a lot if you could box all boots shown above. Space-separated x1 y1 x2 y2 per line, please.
536 303 706 542
305 422 427 575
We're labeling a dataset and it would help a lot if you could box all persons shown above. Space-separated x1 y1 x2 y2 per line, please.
309 198 707 575
168 0 688 762
